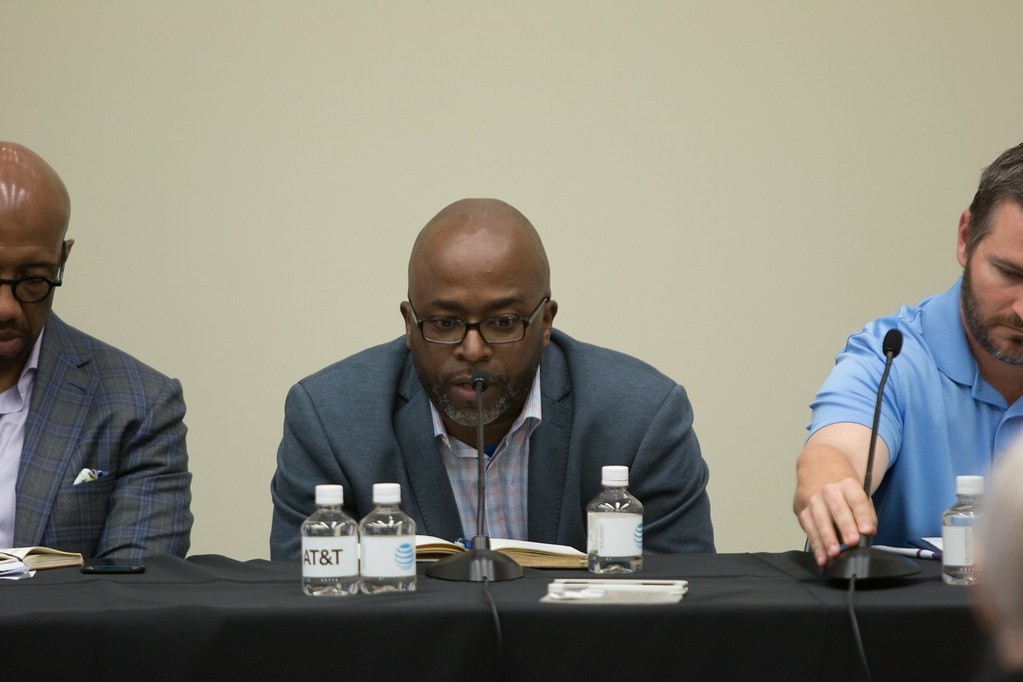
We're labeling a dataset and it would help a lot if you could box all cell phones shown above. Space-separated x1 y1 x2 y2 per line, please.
81 557 145 574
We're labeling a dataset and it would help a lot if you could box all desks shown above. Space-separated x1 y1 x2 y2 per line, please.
0 556 1023 682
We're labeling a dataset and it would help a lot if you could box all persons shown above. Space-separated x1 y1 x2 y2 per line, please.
270 198 717 560
793 142 1023 566
0 141 195 563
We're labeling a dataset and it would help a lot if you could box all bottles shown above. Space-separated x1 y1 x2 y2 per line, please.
940 475 986 586
358 482 418 595
299 484 359 597
586 464 643 575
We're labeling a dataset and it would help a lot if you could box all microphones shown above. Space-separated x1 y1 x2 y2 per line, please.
823 328 923 581
425 370 523 582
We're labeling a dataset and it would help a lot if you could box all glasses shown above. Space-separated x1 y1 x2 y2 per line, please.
407 293 551 346
0 241 67 302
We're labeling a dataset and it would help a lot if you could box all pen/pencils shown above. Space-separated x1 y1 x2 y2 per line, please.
871 546 942 560
